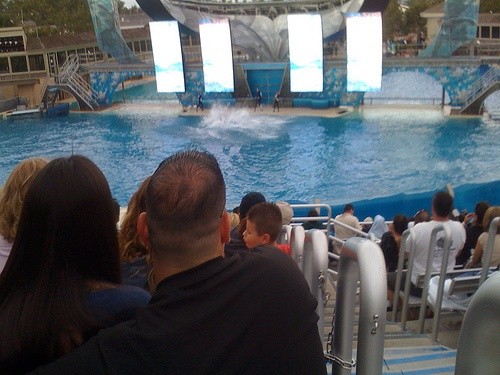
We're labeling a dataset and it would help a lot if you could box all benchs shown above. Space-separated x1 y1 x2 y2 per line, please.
395 264 500 318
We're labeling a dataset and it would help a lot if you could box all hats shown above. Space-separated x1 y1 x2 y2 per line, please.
240 192 267 217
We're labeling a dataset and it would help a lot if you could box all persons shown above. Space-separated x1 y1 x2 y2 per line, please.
366 215 389 246
112 177 157 294
301 210 327 231
273 91 280 111
26 149 328 375
255 88 264 111
225 191 266 257
361 216 374 233
0 157 50 274
328 203 363 268
466 205 500 297
196 90 203 111
385 191 466 320
244 202 292 257
237 31 428 62
0 153 153 375
456 202 490 265
